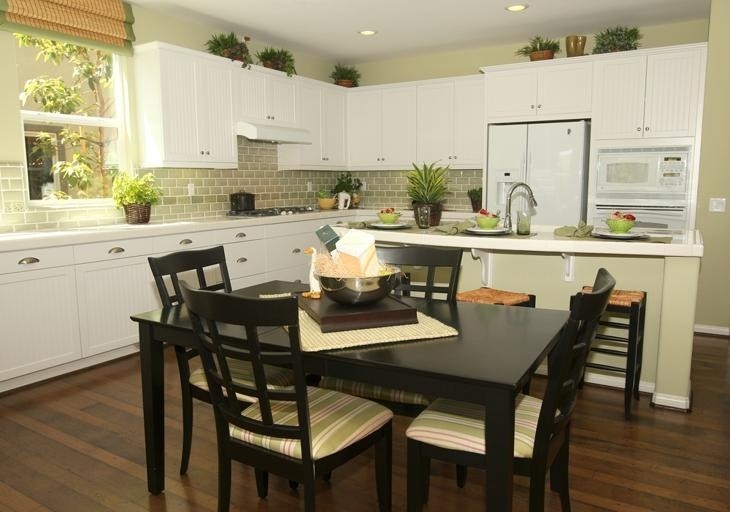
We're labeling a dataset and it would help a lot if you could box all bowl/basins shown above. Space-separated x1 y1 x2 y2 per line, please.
606 217 635 232
315 264 405 308
476 215 502 229
377 211 404 223
318 198 338 210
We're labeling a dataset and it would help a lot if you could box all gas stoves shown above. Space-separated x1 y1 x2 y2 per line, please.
228 206 313 216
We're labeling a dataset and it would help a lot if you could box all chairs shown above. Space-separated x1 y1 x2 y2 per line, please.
144 246 298 474
320 246 466 510
177 277 394 511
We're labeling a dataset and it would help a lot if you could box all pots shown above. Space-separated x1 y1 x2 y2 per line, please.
229 190 255 212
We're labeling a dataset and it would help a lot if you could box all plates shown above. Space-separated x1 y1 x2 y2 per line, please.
371 223 410 229
466 226 511 235
592 231 647 240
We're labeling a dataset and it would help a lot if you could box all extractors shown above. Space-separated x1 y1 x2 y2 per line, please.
235 122 315 145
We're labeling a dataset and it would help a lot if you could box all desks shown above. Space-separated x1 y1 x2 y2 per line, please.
127 279 574 510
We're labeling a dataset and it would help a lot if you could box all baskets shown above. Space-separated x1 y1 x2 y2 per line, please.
123 201 151 225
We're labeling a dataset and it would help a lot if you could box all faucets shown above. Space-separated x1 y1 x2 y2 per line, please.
503 182 537 236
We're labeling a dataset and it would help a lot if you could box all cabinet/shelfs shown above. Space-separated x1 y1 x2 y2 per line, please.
78 226 145 363
342 83 413 172
233 59 295 122
483 57 590 122
588 41 706 143
145 223 268 294
133 41 236 169
413 73 485 170
0 231 78 387
270 207 351 284
279 76 346 172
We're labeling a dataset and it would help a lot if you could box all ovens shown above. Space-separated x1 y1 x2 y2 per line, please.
596 199 687 229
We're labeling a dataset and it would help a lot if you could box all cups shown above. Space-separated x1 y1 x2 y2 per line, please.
337 192 351 210
565 35 588 57
517 211 531 236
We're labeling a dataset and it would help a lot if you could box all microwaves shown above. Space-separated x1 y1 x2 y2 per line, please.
599 154 686 195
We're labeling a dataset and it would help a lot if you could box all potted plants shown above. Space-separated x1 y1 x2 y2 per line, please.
593 26 640 54
401 160 456 228
256 48 298 77
211 30 251 67
330 61 360 89
466 187 481 212
318 174 363 211
514 33 561 61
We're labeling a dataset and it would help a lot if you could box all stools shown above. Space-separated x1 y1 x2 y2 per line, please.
456 285 536 311
580 286 648 418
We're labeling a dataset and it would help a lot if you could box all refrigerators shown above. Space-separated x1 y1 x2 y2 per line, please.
484 119 588 238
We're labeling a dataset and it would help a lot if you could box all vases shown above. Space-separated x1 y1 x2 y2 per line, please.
564 36 586 58
